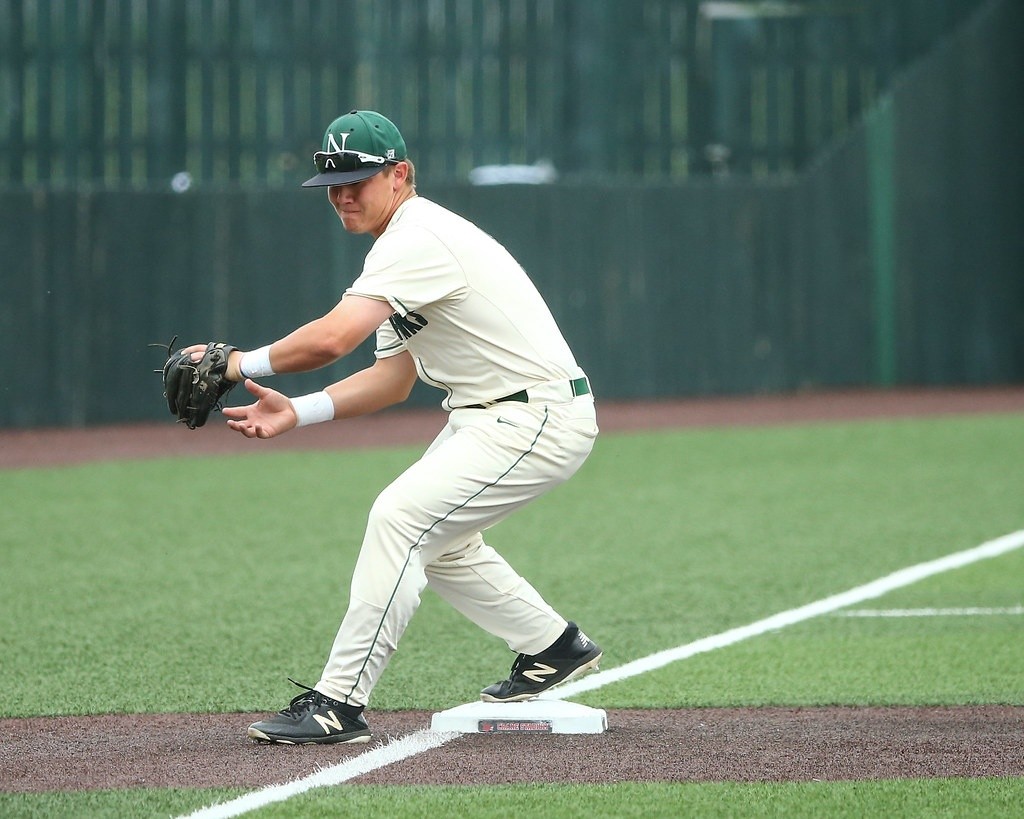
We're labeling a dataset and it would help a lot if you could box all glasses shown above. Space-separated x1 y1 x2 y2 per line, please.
312 149 401 174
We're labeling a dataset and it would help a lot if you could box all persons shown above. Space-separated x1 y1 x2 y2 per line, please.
180 109 604 744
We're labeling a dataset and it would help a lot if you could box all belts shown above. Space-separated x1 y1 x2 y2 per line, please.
464 377 589 409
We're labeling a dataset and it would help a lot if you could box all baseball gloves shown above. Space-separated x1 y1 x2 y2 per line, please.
162 341 240 430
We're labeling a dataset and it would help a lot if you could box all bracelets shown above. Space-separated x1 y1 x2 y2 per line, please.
237 344 276 380
289 391 335 428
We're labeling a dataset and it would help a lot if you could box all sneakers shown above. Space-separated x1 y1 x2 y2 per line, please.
480 621 603 702
248 678 371 745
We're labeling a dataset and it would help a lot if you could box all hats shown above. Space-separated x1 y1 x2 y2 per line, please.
302 110 407 188
